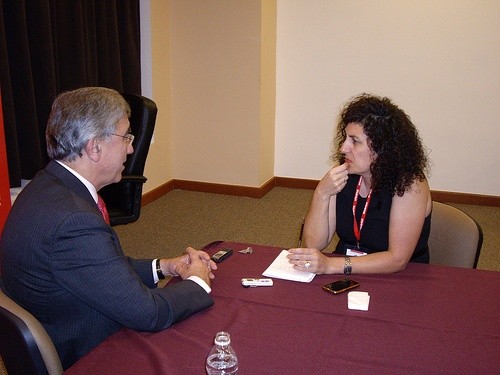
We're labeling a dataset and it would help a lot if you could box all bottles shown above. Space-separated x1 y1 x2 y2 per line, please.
206 330 239 375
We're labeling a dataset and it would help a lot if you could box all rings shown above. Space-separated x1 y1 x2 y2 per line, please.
304 263 310 271
343 178 345 183
305 260 310 265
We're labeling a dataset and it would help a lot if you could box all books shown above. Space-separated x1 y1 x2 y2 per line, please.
262 249 316 283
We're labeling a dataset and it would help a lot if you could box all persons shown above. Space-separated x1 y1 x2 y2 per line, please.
287 93 432 275
0 86 218 375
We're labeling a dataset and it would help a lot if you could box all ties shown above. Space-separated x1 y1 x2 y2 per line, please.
96 193 110 225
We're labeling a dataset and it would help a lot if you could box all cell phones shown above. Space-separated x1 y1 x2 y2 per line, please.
211 248 232 262
321 279 360 294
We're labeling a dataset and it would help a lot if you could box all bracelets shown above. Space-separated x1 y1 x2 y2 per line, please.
156 259 166 280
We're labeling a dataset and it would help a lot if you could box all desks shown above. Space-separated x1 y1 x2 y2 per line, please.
65 238 500 375
10 184 111 226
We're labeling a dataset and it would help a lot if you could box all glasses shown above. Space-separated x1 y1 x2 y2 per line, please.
104 131 134 146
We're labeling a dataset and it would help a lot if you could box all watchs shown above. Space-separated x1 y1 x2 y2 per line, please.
343 256 352 275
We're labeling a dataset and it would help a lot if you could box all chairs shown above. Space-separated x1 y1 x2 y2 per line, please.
431 200 484 270
0 286 64 375
106 89 158 229
